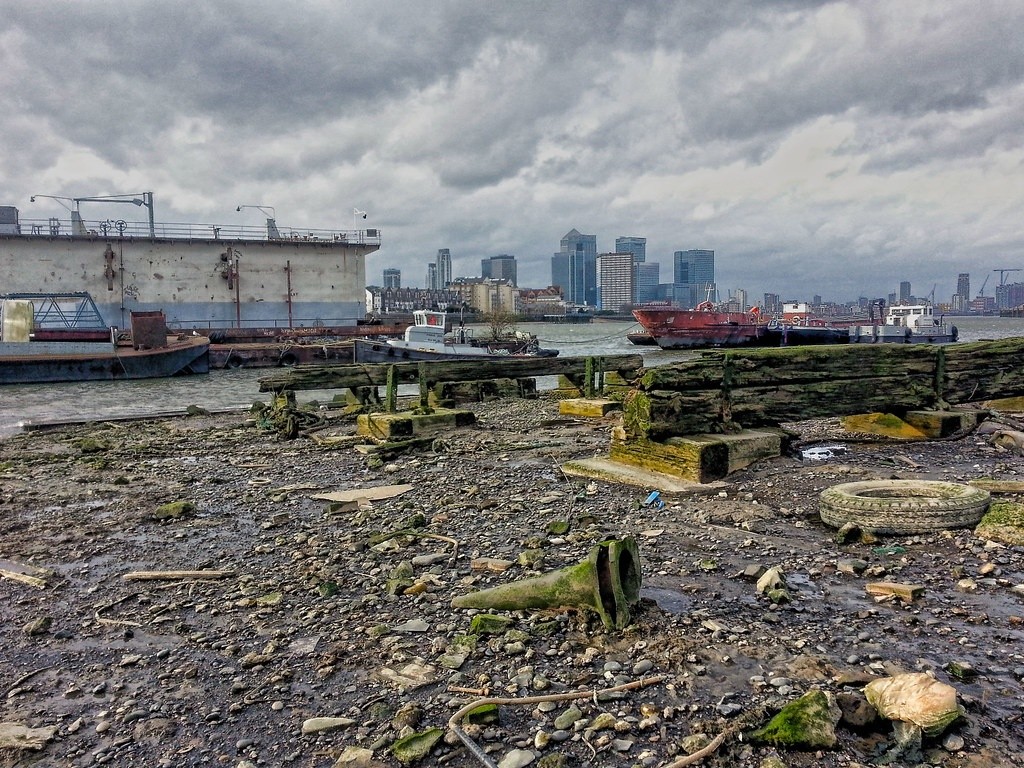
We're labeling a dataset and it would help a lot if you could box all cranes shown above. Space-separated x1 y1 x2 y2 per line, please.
979 275 988 297
993 268 1022 287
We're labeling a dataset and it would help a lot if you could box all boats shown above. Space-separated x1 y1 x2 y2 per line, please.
626 330 657 345
631 281 888 347
0 311 211 383
353 310 559 362
0 187 426 367
848 305 959 344
